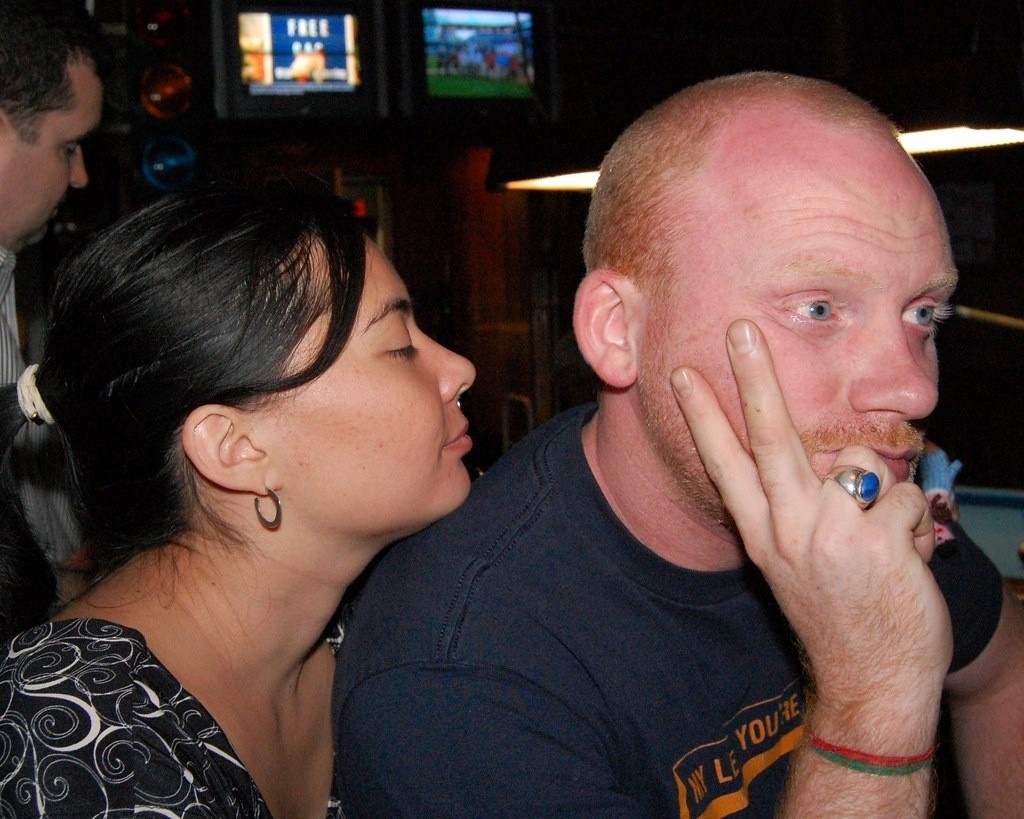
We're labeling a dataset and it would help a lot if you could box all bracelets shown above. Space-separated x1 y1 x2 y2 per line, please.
806 734 936 776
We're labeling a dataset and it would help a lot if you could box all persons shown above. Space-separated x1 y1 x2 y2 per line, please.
0 175 478 819
0 0 125 612
330 73 1024 819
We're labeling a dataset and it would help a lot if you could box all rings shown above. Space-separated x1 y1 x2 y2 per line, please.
823 465 880 510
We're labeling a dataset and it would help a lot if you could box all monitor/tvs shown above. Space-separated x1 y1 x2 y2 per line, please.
413 1 545 112
213 0 386 126
